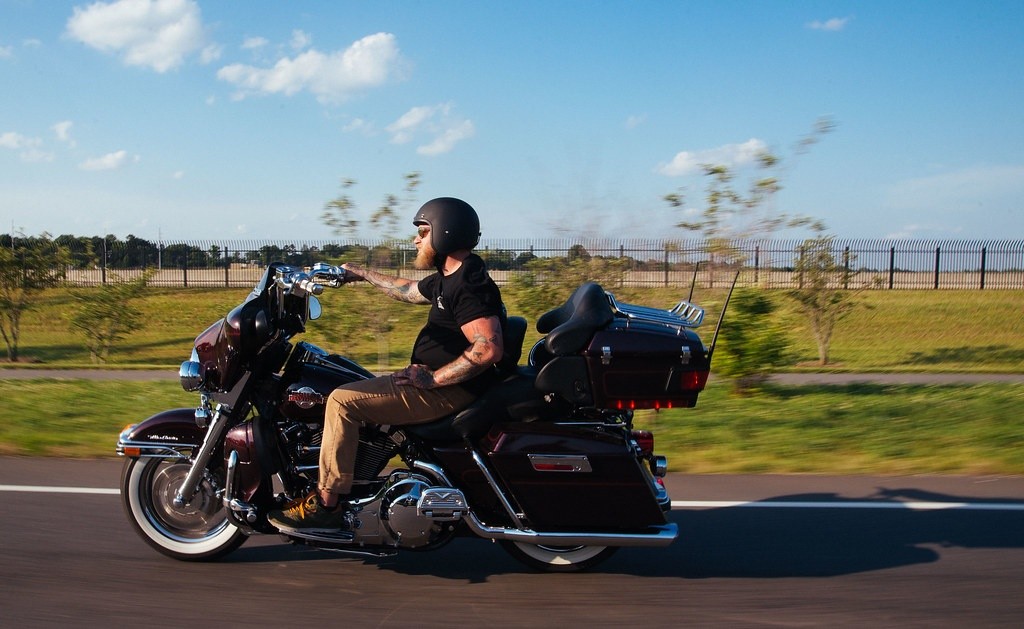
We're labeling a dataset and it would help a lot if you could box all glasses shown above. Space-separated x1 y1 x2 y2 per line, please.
419 227 430 238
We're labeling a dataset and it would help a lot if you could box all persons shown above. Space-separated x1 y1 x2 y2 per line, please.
265 197 508 544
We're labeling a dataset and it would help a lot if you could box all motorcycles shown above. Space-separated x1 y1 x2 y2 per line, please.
116 255 743 574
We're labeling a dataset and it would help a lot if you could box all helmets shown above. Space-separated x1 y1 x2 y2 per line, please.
413 197 480 256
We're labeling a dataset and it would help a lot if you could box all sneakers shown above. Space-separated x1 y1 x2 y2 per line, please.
270 489 343 533
